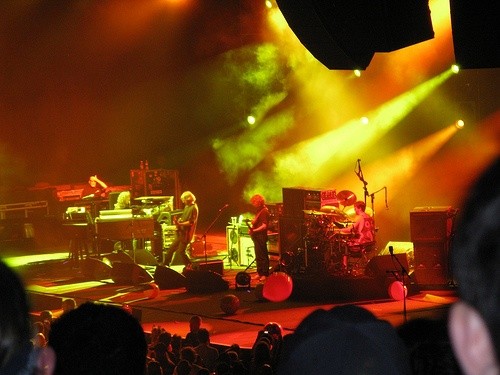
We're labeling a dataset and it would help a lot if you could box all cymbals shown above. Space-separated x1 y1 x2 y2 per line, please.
303 209 330 216
336 190 356 206
320 206 347 222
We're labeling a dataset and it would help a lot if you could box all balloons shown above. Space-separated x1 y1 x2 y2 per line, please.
262 272 292 303
255 284 268 300
144 283 159 298
390 281 407 299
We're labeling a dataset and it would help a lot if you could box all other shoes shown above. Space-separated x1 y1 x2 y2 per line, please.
258 276 266 281
251 274 260 280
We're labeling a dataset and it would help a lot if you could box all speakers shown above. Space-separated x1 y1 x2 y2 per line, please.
366 253 412 298
154 265 185 289
413 241 451 285
81 257 111 279
111 261 154 283
225 225 239 263
183 268 226 293
239 234 257 266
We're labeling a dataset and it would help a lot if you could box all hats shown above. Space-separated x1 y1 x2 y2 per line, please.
274 305 411 375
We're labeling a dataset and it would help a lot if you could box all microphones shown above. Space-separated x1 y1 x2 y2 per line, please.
389 246 393 259
385 202 389 210
219 204 229 212
358 173 368 185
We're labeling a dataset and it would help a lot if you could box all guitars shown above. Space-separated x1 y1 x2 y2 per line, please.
173 216 187 243
246 220 256 241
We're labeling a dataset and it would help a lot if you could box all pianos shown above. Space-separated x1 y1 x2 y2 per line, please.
133 195 175 264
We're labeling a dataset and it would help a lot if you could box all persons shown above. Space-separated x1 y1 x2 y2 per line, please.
164 191 198 266
0 157 500 375
81 175 107 199
116 191 131 210
336 201 375 271
248 194 271 281
